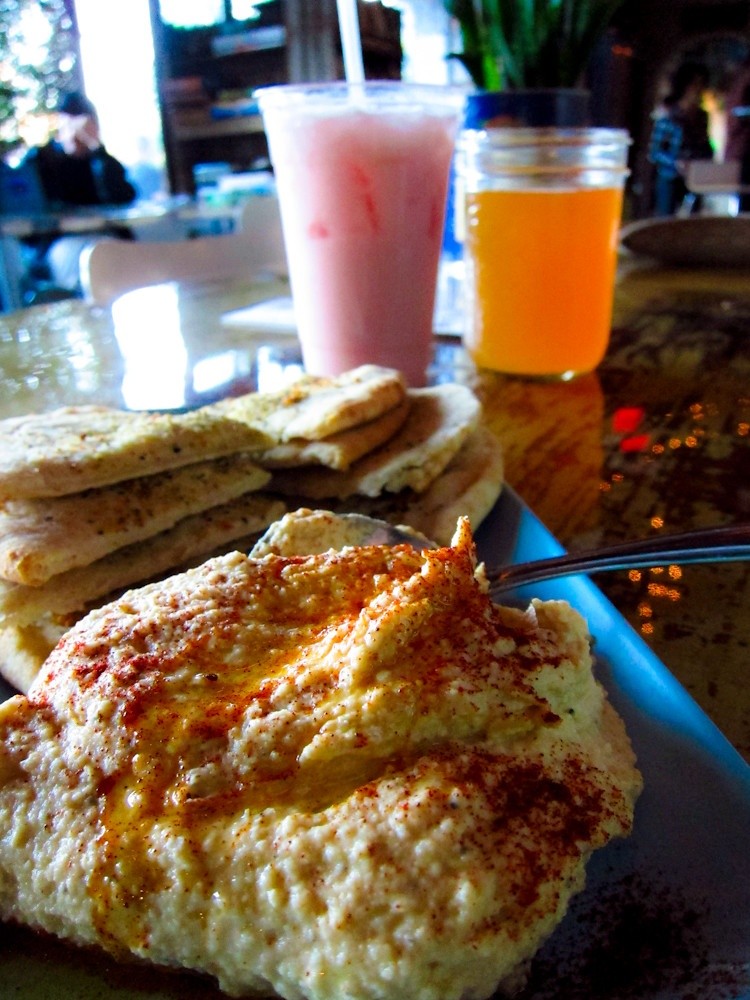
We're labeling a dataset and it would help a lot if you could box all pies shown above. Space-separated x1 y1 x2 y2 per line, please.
0 403 288 695
199 363 505 546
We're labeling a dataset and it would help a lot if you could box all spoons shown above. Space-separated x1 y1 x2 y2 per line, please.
248 514 749 597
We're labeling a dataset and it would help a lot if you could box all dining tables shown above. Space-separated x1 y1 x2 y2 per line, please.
0 269 750 1000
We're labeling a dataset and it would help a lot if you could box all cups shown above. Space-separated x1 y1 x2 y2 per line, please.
457 128 635 379
254 84 468 388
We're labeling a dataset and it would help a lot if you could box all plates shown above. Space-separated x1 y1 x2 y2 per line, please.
0 479 750 1000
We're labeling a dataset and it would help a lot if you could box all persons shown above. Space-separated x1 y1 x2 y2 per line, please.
0 88 136 318
645 62 714 218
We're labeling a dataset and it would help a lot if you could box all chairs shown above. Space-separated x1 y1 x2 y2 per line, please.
78 196 288 298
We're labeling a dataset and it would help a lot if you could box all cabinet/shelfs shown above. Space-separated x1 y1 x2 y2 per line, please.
150 0 402 196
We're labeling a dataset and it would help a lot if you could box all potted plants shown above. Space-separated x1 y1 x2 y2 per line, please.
441 0 593 126
